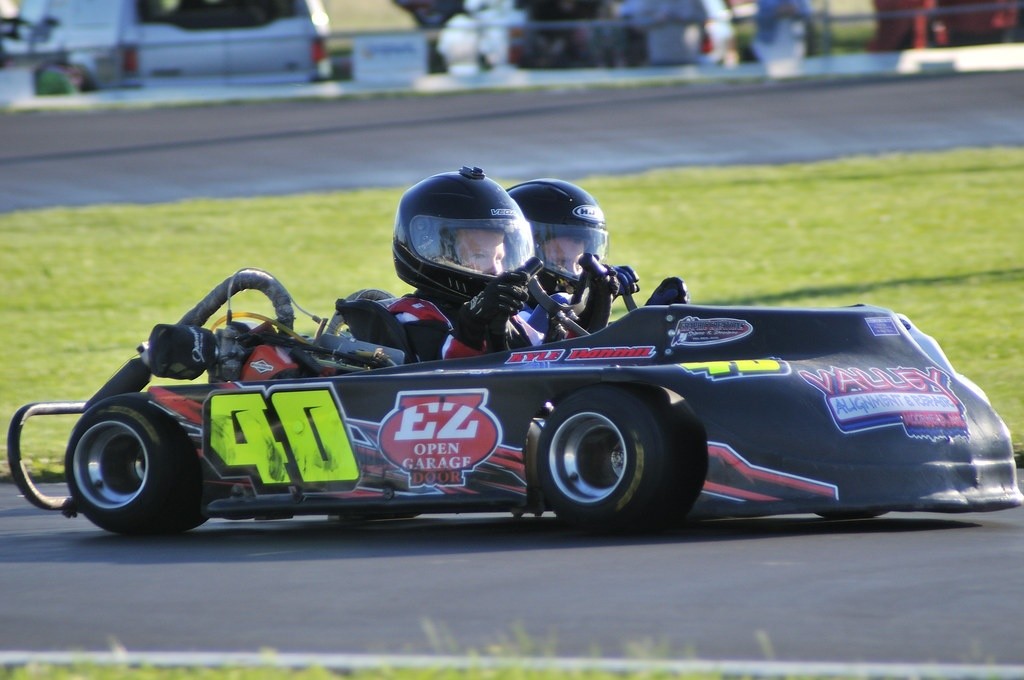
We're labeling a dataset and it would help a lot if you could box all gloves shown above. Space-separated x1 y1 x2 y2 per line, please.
578 265 619 327
647 277 688 306
615 265 640 295
450 270 528 351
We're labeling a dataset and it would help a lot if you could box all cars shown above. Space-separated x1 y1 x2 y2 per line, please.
0 1 353 108
434 0 1024 75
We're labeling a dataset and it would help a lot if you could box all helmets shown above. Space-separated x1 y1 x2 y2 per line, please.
392 165 532 303
504 178 608 312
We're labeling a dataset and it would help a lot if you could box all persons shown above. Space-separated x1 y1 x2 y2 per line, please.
388 166 690 360
392 0 1017 68
